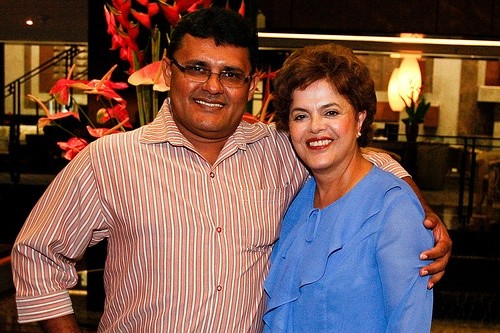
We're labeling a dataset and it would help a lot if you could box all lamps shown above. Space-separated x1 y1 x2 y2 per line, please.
386 55 423 113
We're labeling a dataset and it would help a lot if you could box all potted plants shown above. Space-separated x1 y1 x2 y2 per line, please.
398 89 431 143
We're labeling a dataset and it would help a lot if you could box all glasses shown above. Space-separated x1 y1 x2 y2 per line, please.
168 56 258 88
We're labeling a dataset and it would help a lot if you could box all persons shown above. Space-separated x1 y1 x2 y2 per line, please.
252 45 438 333
4 8 451 333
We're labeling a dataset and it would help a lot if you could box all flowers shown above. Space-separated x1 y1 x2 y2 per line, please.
25 0 248 161
